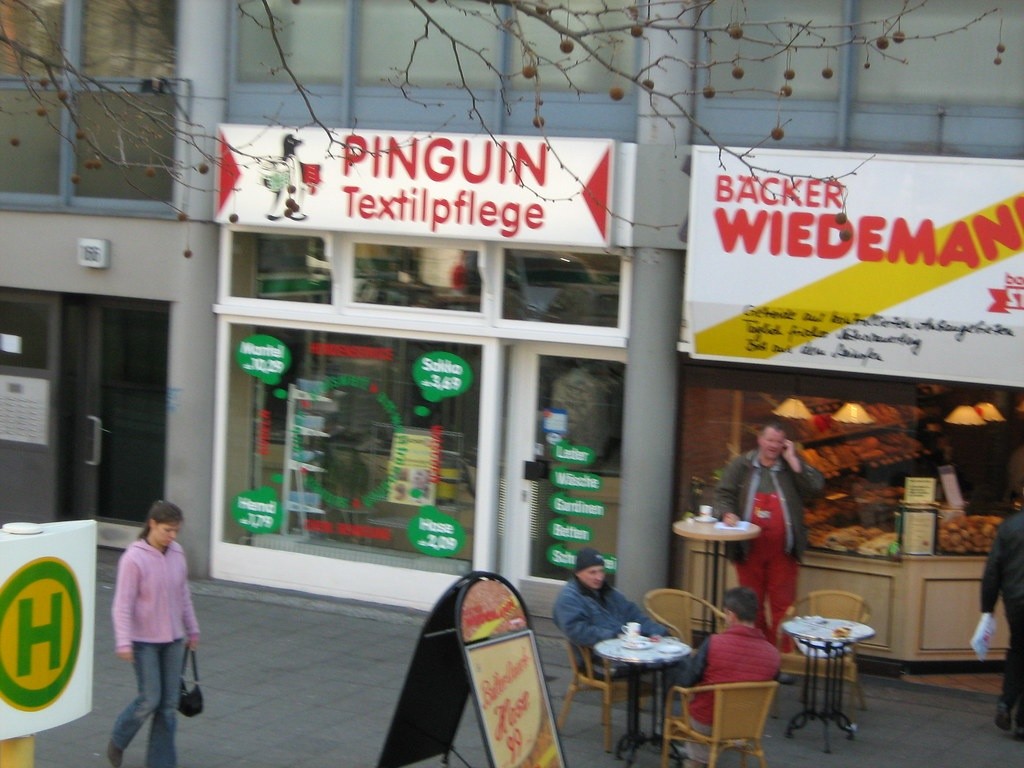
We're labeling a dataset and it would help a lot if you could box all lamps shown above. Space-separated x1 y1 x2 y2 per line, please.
943 404 987 427
771 395 813 421
831 402 876 426
974 401 1006 424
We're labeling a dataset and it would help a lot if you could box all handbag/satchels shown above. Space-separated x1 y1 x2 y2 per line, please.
178 644 203 717
971 610 997 660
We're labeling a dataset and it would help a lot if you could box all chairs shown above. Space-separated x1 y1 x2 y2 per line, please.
660 681 779 768
771 589 873 718
644 588 728 660
557 631 653 753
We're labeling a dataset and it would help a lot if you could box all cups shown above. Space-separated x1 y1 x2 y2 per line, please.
700 505 713 518
620 622 641 636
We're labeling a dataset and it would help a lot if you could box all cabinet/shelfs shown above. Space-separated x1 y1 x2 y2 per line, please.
788 396 946 488
279 383 333 534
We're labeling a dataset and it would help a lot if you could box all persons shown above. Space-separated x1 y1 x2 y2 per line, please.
670 585 782 768
107 500 201 768
552 546 693 755
713 422 819 685
979 447 1024 743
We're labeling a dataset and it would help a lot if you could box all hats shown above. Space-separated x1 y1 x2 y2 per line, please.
576 545 605 571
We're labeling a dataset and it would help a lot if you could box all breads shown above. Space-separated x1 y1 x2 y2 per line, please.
936 515 1006 554
803 474 905 556
800 403 923 473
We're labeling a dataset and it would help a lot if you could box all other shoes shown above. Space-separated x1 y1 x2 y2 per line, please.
779 670 795 685
1015 726 1024 740
109 736 122 768
996 701 1011 732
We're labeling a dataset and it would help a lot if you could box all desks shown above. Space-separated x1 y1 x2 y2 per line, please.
593 638 692 768
780 619 877 754
366 517 474 548
333 503 379 544
673 519 762 635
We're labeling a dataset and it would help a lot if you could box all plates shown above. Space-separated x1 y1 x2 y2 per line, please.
622 635 648 642
694 516 717 523
623 642 652 650
657 644 685 653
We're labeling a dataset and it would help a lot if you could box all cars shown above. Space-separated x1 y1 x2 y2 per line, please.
508 250 618 319
